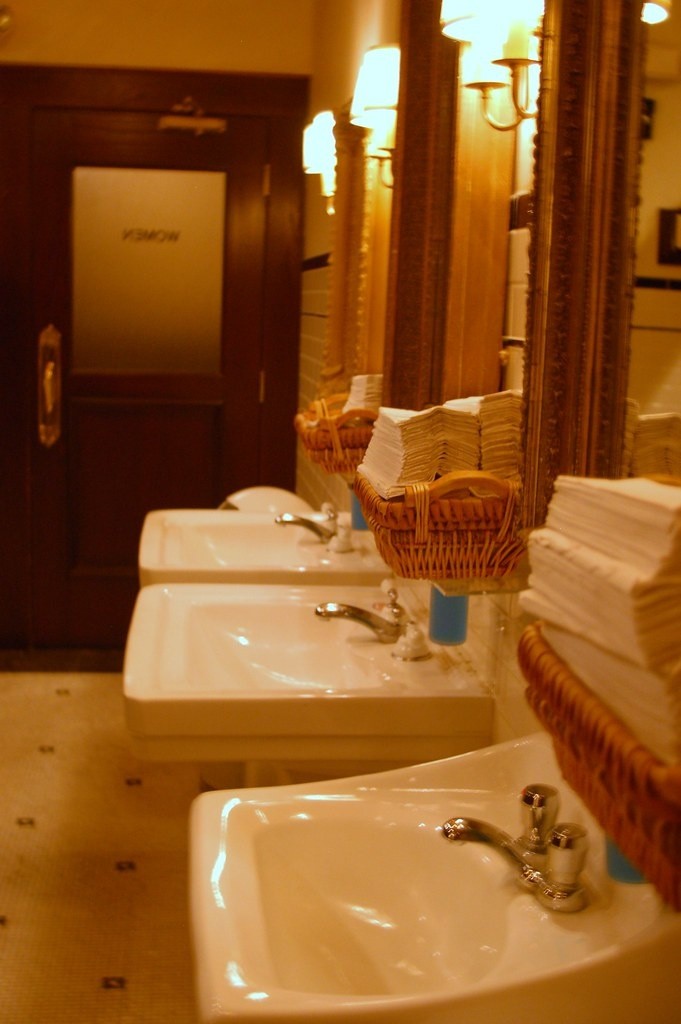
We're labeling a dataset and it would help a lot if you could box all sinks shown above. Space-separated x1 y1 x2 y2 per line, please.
188 726 681 1023
137 510 397 587
122 581 497 759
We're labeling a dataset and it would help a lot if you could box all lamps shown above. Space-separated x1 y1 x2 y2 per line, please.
301 111 338 216
350 43 399 190
437 0 543 131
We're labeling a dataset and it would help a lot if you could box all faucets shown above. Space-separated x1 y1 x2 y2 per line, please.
272 503 338 540
440 783 558 891
308 595 405 643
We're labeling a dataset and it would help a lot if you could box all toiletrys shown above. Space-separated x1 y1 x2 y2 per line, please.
350 492 371 534
430 589 468 648
603 829 650 883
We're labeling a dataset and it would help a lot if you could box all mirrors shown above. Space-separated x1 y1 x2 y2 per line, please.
498 38 540 393
625 0 680 487
